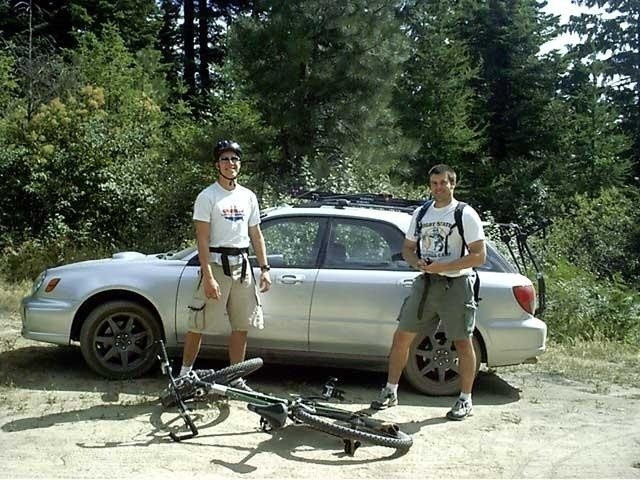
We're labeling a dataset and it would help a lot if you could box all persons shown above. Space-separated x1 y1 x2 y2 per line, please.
178 141 272 391
371 164 486 421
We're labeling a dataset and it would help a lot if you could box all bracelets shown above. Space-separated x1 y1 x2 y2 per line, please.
260 264 271 272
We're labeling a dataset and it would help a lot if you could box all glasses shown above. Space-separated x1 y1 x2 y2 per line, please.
219 155 240 163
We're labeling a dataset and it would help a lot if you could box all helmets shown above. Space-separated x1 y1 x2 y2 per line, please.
213 138 245 161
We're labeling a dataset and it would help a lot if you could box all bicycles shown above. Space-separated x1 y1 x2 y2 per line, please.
156 338 414 457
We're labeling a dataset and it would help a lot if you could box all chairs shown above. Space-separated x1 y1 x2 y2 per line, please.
326 242 347 266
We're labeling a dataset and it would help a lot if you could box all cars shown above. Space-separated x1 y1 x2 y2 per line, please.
19 186 548 396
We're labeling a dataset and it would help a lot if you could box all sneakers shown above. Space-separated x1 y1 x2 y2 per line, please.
445 398 472 421
370 387 399 410
226 379 253 391
